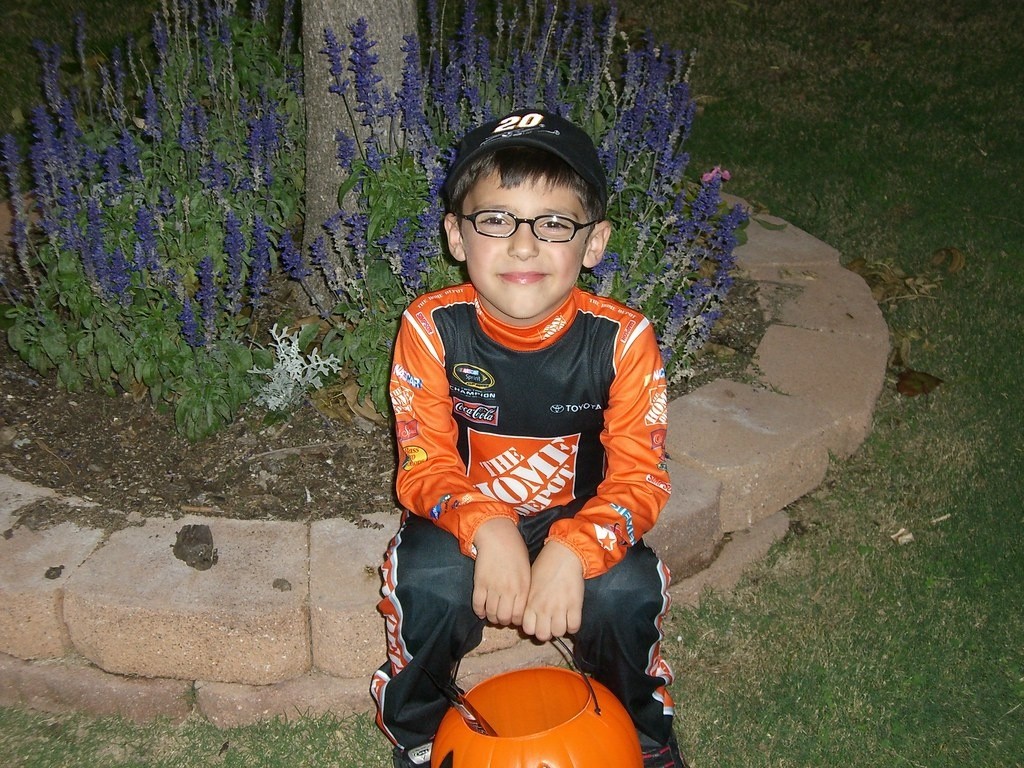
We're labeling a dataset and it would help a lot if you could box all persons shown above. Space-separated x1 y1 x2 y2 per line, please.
371 108 690 768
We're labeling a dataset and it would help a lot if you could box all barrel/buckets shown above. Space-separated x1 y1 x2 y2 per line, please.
430 615 643 768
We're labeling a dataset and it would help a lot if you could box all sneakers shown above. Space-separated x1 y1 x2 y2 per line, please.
393 735 452 768
640 729 689 768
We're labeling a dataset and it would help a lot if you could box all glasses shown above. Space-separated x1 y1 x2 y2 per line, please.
455 210 602 243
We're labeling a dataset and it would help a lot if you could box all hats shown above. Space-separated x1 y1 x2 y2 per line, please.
444 109 609 218
